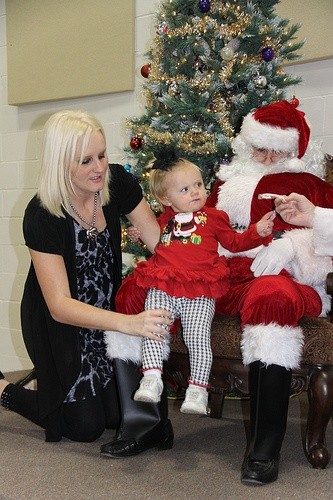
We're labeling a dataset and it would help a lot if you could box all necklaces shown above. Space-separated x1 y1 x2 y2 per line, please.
67 191 101 239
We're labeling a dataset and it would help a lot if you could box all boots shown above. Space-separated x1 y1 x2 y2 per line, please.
239 326 302 484
98 331 175 459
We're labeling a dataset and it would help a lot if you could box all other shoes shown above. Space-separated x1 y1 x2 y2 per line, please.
0 369 37 387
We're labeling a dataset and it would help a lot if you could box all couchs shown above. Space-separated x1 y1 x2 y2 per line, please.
171 154 333 471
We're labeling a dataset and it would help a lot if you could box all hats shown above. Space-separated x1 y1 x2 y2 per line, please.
239 100 311 172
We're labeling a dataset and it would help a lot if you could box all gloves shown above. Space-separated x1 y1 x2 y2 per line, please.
250 237 294 277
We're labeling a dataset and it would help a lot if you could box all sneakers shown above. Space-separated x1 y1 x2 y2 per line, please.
178 386 209 415
132 375 162 404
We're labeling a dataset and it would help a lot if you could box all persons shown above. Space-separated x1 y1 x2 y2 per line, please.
0 109 172 444
273 192 332 258
98 101 326 485
133 157 278 415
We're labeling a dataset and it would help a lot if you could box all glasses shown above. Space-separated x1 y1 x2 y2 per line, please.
250 149 285 163
